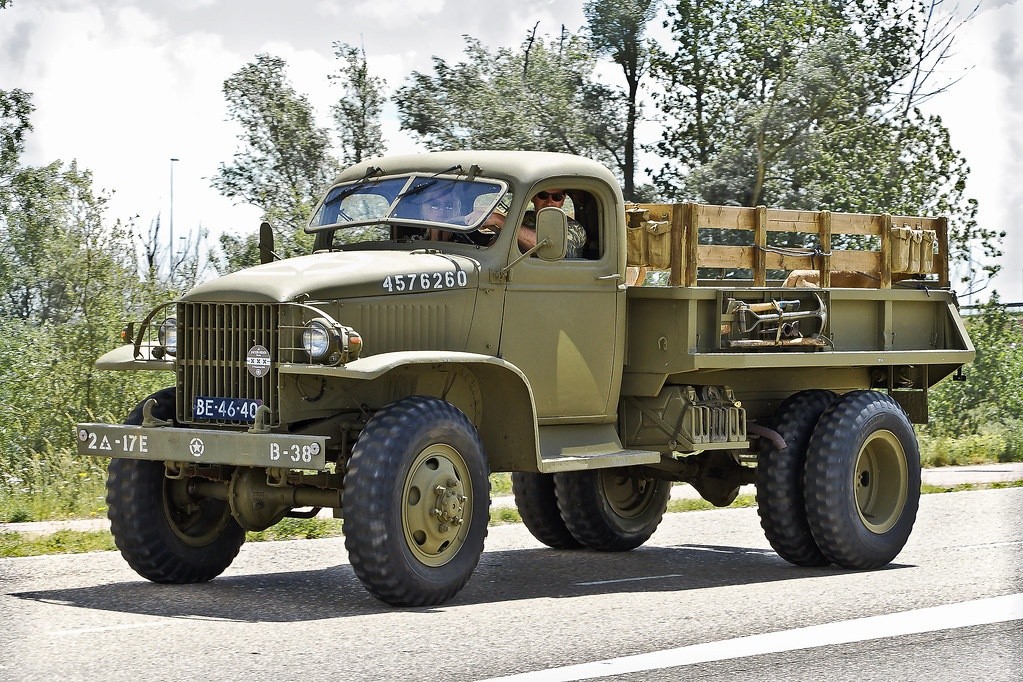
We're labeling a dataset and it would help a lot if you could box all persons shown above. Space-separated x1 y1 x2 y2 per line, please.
464 185 587 260
406 189 466 241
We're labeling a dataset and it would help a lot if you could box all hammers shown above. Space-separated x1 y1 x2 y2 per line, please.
728 333 834 350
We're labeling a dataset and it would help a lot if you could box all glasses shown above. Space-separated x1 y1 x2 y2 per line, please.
430 200 454 211
537 191 566 201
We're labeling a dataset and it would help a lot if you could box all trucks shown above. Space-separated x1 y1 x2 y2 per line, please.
76 150 977 606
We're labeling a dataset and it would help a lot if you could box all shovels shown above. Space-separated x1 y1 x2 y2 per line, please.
738 293 829 338
